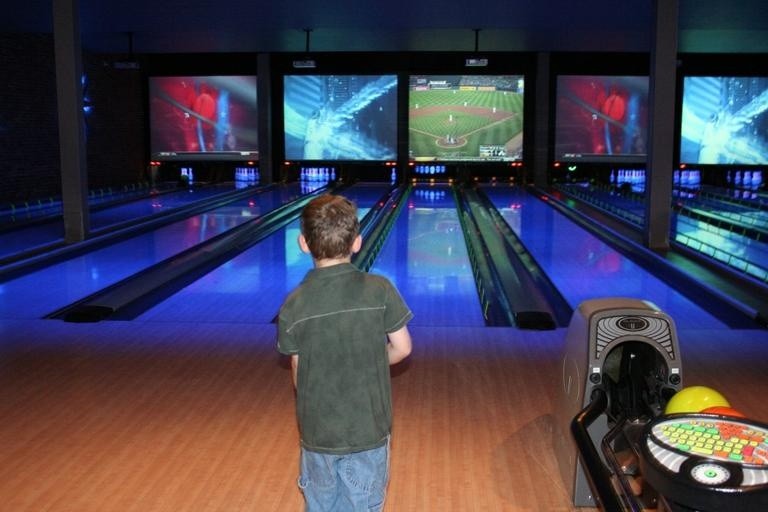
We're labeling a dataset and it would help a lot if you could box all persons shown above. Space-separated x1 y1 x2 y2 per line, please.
276 191 415 512
